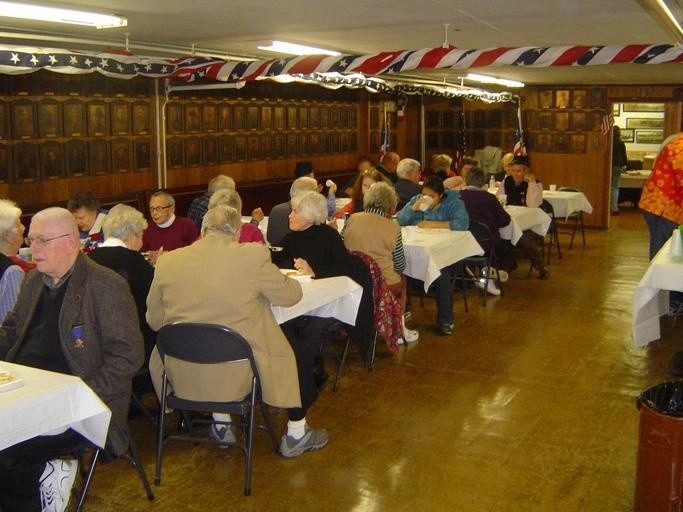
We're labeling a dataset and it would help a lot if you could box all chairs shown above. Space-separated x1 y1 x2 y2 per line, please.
2 273 278 510
210 189 593 390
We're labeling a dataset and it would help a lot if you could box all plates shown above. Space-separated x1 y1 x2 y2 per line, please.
0 371 22 392
277 268 311 281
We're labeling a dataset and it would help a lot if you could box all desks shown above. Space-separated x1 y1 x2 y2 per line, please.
614 168 655 212
628 223 682 378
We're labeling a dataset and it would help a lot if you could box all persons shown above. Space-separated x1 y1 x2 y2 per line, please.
0 199 145 511
68 174 350 456
638 131 683 321
297 153 550 343
610 126 629 216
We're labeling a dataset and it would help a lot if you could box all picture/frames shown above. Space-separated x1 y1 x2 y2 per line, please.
416 87 608 158
0 92 405 183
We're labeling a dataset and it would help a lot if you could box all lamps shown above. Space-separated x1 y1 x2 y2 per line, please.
455 73 527 90
0 0 129 33
255 37 344 58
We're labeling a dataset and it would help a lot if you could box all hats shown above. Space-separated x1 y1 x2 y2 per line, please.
507 155 531 168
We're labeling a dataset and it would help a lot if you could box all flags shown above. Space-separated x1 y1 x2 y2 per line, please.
376 100 391 164
512 96 530 169
454 80 467 174
600 105 616 136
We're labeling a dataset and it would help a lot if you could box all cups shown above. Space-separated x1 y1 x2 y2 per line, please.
669 228 683 257
406 225 416 239
18 247 34 263
418 194 434 212
549 184 557 192
325 179 335 187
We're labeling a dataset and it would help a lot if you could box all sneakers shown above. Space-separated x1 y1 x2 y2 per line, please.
435 319 456 336
207 423 238 449
539 265 552 281
277 422 332 460
475 276 503 297
481 264 509 283
37 457 81 512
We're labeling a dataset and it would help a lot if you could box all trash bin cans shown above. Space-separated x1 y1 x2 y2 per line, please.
634 381 683 512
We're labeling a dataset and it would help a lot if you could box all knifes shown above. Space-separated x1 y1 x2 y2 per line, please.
264 244 283 252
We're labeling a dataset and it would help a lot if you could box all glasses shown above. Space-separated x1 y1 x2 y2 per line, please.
149 204 172 212
23 233 72 247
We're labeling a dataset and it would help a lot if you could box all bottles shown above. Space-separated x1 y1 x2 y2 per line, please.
489 174 496 191
391 215 398 224
342 212 349 223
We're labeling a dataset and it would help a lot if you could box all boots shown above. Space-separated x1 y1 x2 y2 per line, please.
393 313 421 346
